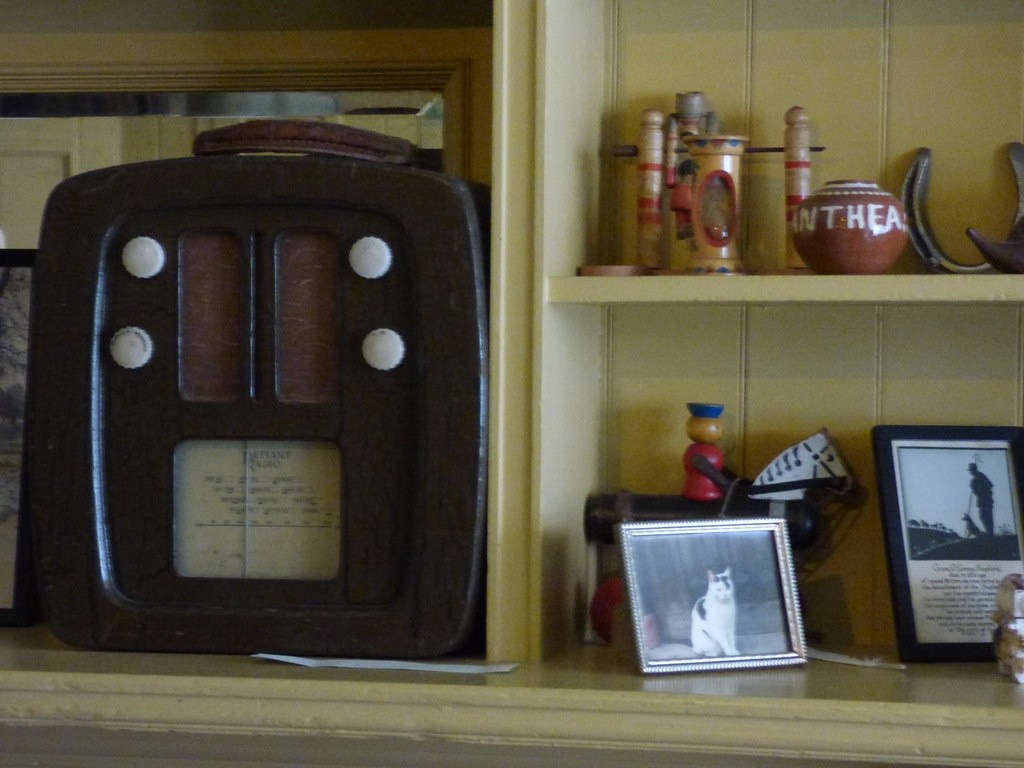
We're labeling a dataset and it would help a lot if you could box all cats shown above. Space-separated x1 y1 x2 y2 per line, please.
689 567 741 657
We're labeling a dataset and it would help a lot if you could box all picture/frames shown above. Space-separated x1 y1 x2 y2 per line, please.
614 517 807 677
872 425 1023 664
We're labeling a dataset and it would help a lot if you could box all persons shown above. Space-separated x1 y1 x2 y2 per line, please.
966 463 994 537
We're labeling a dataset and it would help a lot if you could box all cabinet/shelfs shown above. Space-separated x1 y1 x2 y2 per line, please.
0 1 1024 767
